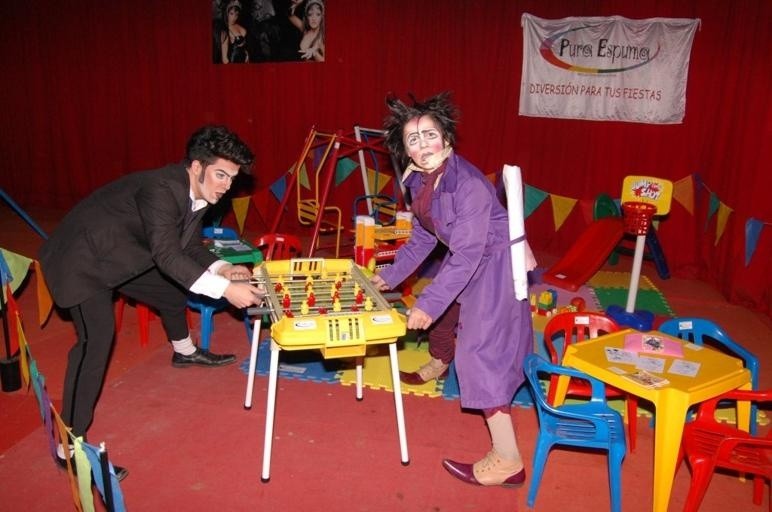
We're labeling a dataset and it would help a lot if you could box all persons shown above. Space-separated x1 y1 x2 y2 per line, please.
368 90 533 487
220 0 325 64
37 124 266 484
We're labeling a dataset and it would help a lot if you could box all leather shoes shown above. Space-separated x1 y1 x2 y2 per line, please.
54 449 129 483
172 345 235 366
398 360 449 383
443 455 526 487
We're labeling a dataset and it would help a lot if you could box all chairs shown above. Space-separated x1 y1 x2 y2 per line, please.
650 317 759 434
673 389 772 512
523 354 627 512
545 310 640 453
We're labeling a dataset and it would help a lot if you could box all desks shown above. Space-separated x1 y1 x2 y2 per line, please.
551 330 752 512
